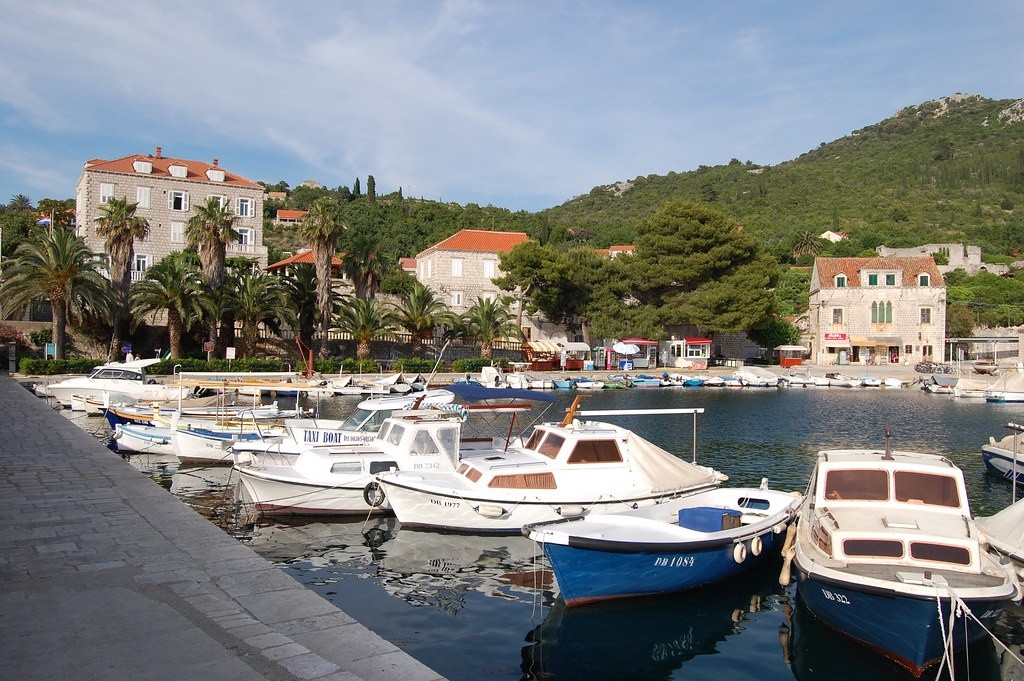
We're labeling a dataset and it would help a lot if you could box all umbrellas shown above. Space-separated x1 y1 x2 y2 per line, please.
561 347 567 372
612 342 640 363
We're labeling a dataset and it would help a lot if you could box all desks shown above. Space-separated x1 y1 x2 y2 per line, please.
680 506 743 533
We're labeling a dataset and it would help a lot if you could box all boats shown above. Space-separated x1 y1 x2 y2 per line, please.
337 334 902 394
229 340 555 517
981 430 1024 487
796 419 1019 678
372 393 729 533
974 497 1024 610
522 477 803 610
33 332 339 466
922 375 958 394
956 347 1024 404
